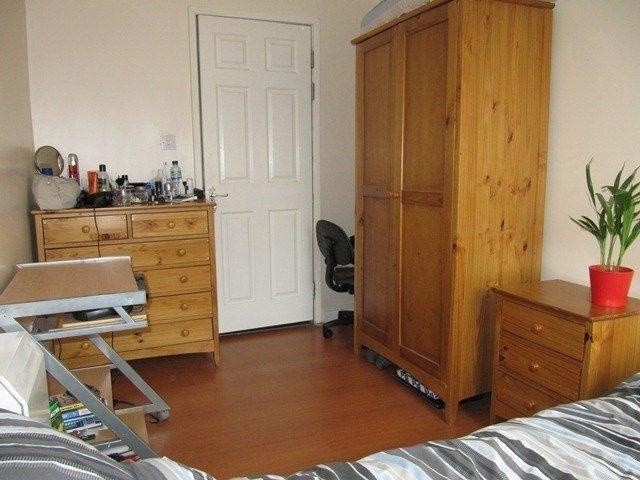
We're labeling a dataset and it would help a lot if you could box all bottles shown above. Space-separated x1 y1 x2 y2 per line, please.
69 153 194 204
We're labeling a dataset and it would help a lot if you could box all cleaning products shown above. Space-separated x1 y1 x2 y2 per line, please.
98 163 109 191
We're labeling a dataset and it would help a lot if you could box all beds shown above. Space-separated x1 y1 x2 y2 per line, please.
0 373 640 480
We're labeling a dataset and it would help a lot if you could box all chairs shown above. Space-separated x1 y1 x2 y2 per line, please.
316 218 355 338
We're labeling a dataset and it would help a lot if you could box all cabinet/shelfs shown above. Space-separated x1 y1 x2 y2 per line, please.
30 201 221 368
350 0 555 427
489 279 640 428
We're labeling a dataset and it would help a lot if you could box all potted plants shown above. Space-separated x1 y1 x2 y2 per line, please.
567 153 640 307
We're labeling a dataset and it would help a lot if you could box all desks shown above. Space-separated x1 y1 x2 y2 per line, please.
0 255 173 459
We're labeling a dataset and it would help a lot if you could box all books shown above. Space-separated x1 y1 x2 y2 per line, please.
48 384 142 462
